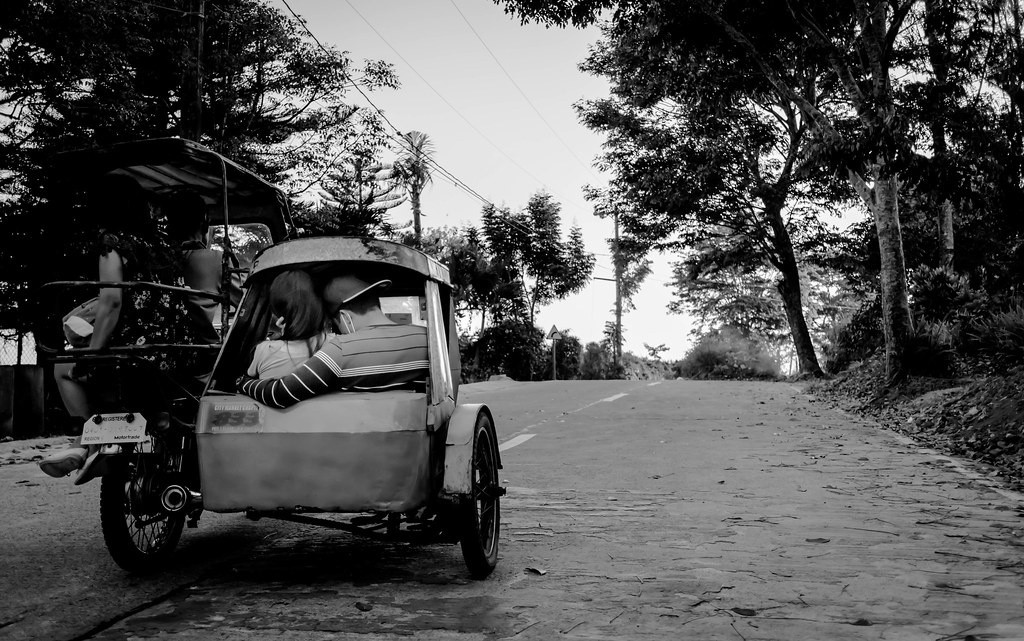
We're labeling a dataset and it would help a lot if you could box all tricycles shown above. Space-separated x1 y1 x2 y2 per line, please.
33 138 507 579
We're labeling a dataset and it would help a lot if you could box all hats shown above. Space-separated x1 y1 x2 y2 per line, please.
323 275 392 318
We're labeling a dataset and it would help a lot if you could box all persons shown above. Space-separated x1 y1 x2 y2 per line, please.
233 270 427 410
36 172 200 485
162 187 228 345
247 271 340 380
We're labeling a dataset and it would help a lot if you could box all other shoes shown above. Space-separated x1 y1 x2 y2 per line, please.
38 447 87 478
73 450 102 485
